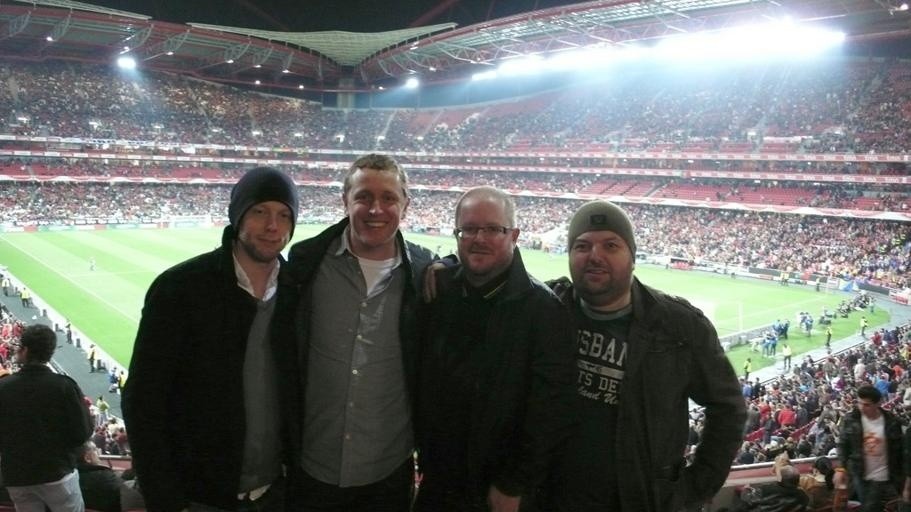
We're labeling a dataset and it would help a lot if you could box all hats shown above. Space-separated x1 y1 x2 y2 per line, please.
229 168 298 242
568 200 637 264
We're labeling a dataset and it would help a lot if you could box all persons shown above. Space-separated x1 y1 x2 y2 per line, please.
421 200 748 512
122 167 299 512
288 155 441 511
683 292 911 512
414 185 578 512
0 269 148 511
0 42 911 300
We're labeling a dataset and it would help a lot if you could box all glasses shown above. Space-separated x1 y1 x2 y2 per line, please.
454 225 514 239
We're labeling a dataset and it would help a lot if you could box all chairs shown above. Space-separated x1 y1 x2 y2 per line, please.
744 392 905 444
